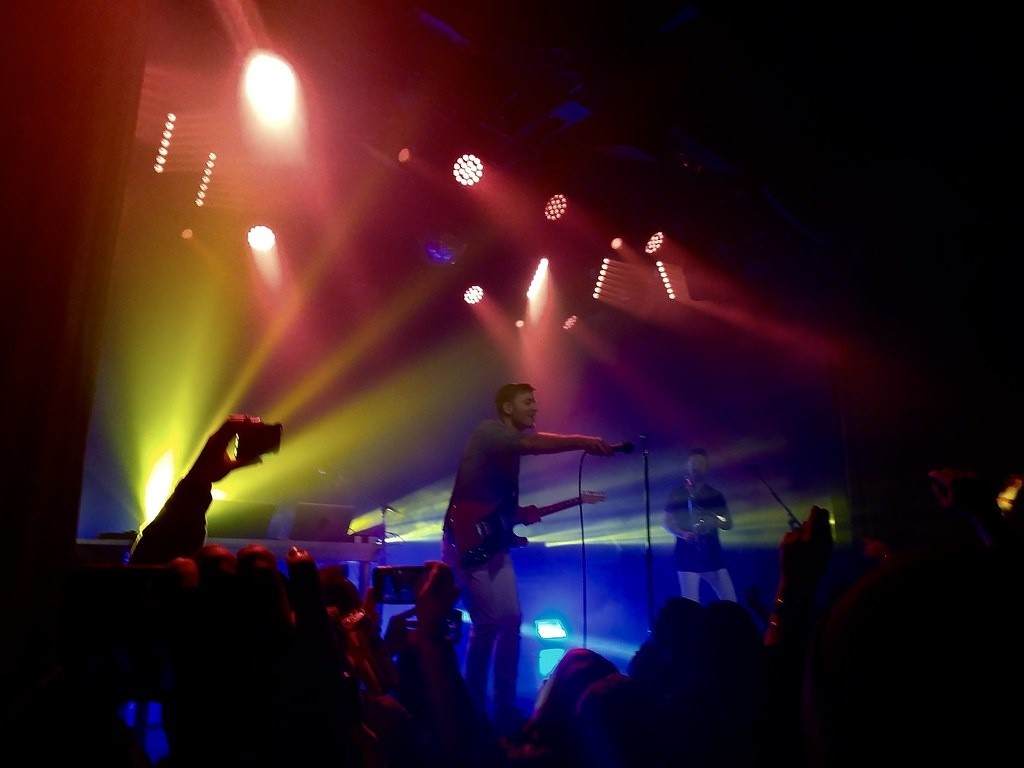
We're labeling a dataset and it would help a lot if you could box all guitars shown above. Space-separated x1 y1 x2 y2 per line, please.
445 488 609 572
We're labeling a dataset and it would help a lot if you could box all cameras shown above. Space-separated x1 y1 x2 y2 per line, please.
235 423 282 459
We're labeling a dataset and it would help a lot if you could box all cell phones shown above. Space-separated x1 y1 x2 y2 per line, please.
372 564 430 606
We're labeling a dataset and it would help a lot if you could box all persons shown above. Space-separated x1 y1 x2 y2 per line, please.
0 417 1023 768
443 383 612 721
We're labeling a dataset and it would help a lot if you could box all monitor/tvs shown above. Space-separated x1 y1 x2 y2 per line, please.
208 500 274 539
289 502 356 541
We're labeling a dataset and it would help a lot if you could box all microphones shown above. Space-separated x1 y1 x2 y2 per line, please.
610 441 636 454
